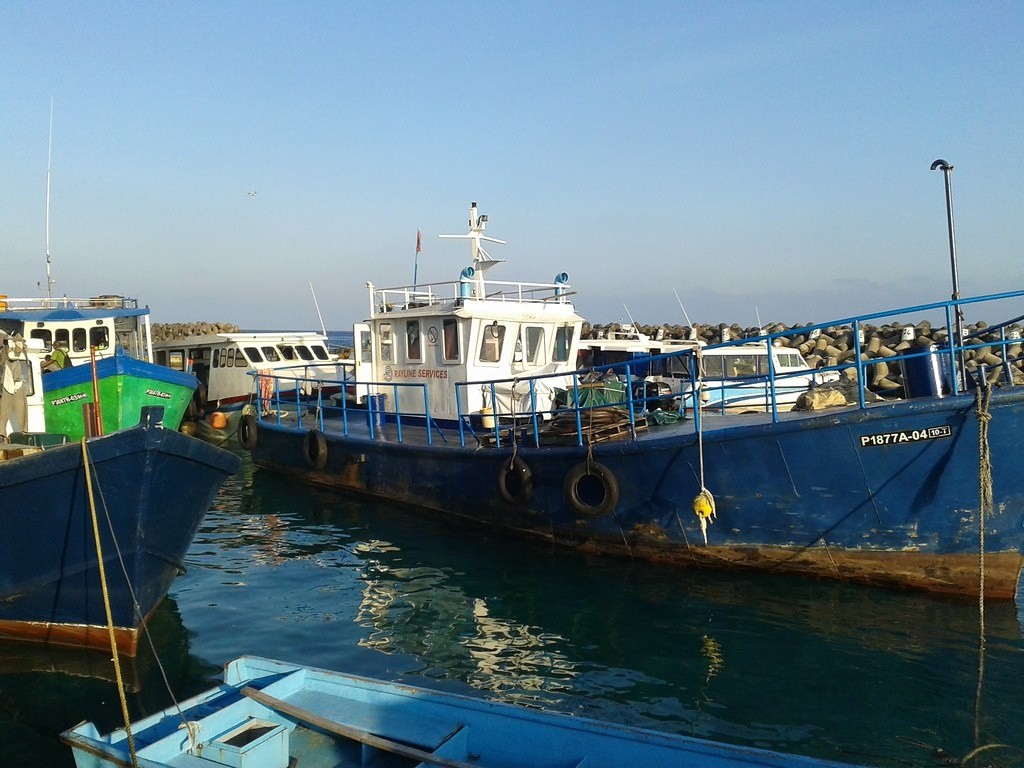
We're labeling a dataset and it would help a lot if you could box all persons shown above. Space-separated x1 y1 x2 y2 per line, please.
40 341 69 373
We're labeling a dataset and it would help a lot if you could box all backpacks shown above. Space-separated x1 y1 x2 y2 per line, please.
56 349 72 369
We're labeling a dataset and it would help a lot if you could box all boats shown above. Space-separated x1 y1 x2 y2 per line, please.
240 159 1024 607
54 654 875 768
1 93 198 445
148 282 356 440
1 299 241 701
579 289 842 417
